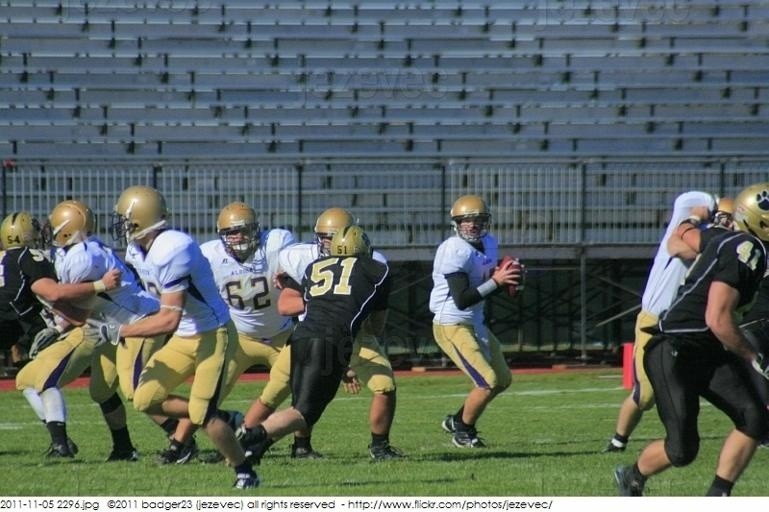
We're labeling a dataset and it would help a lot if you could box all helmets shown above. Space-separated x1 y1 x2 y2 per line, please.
450 194 492 240
0 210 40 249
713 197 734 228
731 181 769 242
43 199 93 248
216 201 261 259
109 184 169 245
314 207 356 259
330 224 374 258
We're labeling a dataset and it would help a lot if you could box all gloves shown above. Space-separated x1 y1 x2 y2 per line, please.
84 312 124 348
29 327 60 360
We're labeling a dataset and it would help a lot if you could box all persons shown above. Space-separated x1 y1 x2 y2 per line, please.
0 211 122 351
238 225 390 466
82 185 261 490
15 317 97 462
427 195 527 451
614 182 769 497
161 201 295 467
49 198 195 464
243 207 400 461
605 191 734 454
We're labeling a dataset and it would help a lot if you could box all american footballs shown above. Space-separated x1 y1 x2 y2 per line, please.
498 255 523 298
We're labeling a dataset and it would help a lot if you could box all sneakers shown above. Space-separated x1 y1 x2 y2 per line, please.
106 448 138 462
440 413 459 434
160 437 196 467
156 448 175 470
600 443 624 455
367 439 401 460
225 427 248 467
451 428 486 448
613 464 643 496
40 444 73 460
231 472 259 490
287 443 323 460
66 435 78 454
